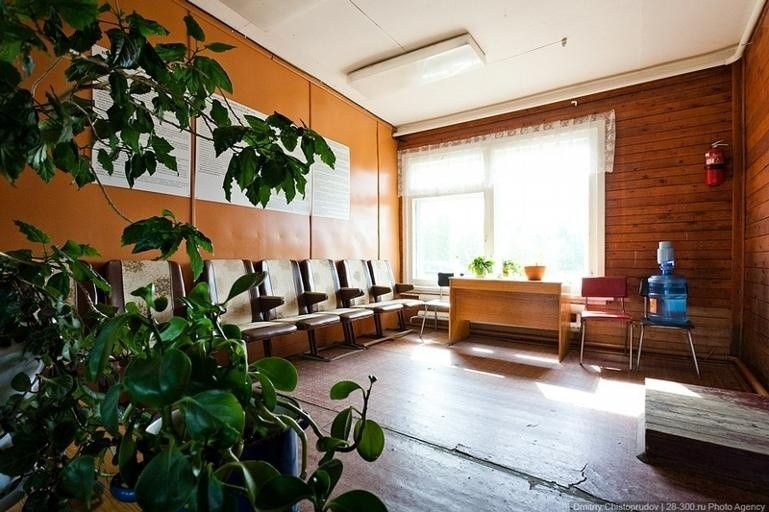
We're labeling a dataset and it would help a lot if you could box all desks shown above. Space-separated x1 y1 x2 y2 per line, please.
447 277 570 362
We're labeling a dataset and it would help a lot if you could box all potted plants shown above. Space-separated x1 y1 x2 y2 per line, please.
467 256 493 278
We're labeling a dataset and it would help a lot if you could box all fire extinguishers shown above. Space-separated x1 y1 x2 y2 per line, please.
704 138 729 187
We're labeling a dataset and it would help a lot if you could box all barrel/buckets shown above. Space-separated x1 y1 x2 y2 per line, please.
648 262 689 325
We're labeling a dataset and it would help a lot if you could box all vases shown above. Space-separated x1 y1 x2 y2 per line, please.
525 266 546 281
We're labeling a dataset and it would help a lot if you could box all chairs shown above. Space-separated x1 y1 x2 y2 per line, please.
28 258 97 313
580 276 634 371
636 277 701 380
104 260 188 321
419 273 454 339
204 258 424 359
